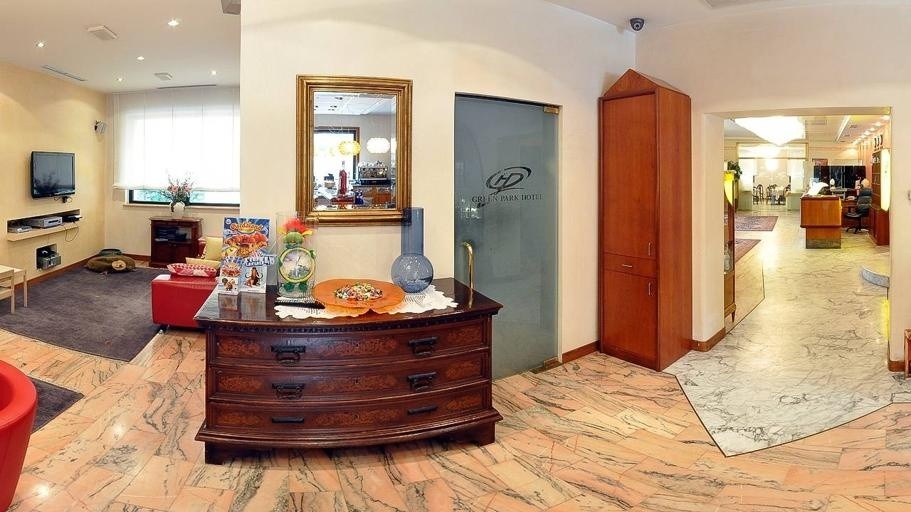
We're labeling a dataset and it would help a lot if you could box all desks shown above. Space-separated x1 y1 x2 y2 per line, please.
0 265 28 315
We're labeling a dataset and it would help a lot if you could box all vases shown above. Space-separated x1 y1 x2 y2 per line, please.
391 207 434 294
169 201 186 220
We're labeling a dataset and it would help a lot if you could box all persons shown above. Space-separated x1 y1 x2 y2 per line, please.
246 267 259 287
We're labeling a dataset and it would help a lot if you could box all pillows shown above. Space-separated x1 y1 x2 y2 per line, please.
184 257 221 277
204 238 224 261
167 262 217 279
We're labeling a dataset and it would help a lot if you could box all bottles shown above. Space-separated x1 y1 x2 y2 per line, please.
339 161 347 196
724 243 731 272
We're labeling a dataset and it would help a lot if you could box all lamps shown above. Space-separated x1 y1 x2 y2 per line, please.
94 119 106 134
367 137 390 154
338 128 362 156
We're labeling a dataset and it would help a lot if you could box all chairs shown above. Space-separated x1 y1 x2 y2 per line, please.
753 184 790 205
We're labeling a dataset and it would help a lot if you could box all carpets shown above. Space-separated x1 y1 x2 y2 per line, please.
724 213 779 231
724 238 761 263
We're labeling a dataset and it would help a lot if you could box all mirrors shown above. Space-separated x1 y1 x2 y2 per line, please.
296 75 413 228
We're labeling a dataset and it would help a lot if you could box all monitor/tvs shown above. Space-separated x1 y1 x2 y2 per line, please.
30 151 76 198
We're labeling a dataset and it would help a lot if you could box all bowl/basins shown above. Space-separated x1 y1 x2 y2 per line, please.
324 182 335 188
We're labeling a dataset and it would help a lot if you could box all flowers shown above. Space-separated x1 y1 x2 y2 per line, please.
158 176 197 205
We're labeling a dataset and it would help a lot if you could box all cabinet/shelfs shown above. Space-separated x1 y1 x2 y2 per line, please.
598 68 692 373
349 178 392 204
192 277 503 466
148 215 204 268
724 170 737 316
868 148 889 245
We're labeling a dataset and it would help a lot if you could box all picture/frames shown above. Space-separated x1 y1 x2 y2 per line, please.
873 135 883 147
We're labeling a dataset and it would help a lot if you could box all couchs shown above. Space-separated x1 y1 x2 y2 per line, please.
151 238 224 331
